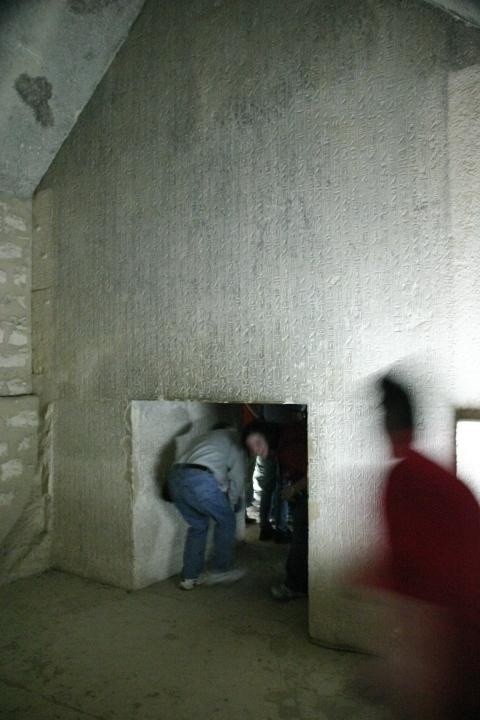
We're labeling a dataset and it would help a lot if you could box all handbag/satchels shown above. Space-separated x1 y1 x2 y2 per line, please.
162 482 175 504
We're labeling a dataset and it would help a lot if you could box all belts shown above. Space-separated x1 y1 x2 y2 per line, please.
170 462 213 474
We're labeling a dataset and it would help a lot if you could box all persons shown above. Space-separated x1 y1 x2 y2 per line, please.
352 377 480 720
167 403 308 601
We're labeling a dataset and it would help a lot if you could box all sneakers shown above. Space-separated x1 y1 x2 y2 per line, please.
178 569 250 590
270 577 308 600
